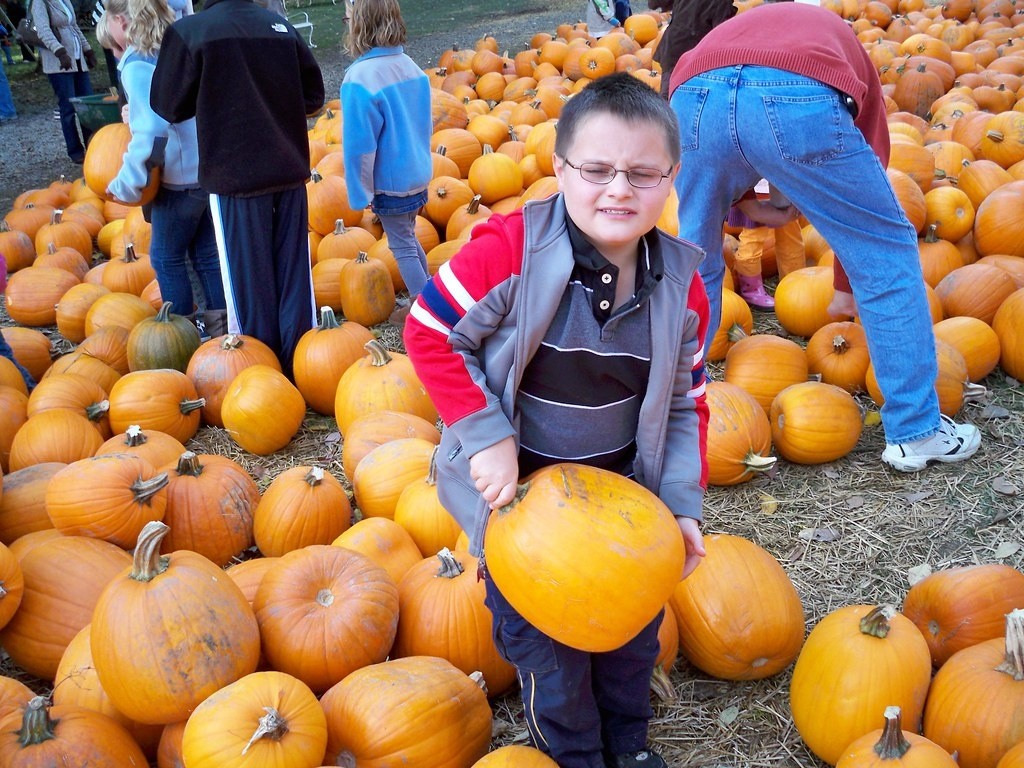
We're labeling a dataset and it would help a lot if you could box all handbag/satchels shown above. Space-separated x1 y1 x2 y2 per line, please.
18 0 61 49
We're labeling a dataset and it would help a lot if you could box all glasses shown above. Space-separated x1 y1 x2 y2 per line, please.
342 16 350 24
563 158 673 189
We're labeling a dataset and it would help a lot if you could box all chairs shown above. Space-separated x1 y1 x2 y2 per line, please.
287 12 317 48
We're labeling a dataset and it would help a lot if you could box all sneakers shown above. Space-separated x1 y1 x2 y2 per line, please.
881 413 982 472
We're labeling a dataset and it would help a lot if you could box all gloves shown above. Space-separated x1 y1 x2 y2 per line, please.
84 49 97 69
55 47 72 71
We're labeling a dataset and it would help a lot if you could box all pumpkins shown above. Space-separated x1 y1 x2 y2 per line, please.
0 0 1024 768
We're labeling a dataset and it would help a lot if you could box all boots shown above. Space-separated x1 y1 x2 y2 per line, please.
389 297 416 326
204 309 228 339
738 272 775 312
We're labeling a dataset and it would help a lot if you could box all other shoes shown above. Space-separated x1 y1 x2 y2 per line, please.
195 318 211 343
611 747 668 768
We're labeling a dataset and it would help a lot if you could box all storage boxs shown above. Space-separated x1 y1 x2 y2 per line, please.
70 91 122 132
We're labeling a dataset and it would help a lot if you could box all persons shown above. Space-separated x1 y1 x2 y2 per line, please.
403 72 710 768
149 0 325 392
340 0 433 342
586 0 793 97
0 0 196 164
668 2 981 472
97 0 234 343
